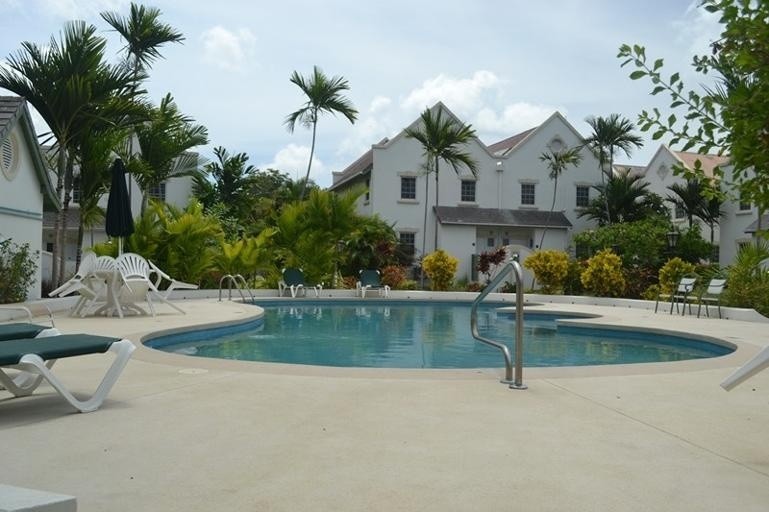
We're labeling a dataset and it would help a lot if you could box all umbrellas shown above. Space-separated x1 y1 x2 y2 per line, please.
106 156 136 256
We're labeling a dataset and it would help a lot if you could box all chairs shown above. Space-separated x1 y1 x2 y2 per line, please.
653 271 730 320
